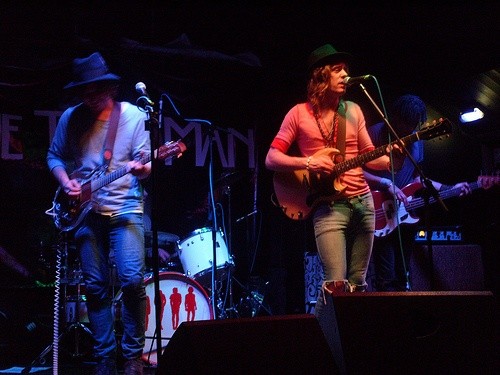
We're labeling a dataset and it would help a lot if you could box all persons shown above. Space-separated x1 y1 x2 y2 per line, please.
264 44 407 339
369 93 470 292
44 51 154 375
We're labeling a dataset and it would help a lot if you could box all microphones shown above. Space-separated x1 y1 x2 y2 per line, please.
343 74 372 86
135 81 153 105
158 99 164 129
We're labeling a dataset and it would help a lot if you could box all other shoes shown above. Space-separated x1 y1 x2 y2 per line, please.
123 359 139 375
94 363 114 375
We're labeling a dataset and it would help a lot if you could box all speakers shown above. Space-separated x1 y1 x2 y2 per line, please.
155 313 343 374
332 288 499 375
408 243 485 292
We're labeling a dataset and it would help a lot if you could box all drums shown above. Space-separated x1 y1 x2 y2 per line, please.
174 224 237 286
60 235 85 285
150 262 178 272
111 268 217 367
150 232 180 262
65 294 90 323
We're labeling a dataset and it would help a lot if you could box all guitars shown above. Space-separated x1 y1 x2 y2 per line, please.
371 174 500 238
52 138 187 232
272 117 455 221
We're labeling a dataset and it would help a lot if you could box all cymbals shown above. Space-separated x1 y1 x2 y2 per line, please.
200 169 238 192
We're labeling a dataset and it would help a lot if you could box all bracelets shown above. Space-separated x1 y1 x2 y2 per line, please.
379 177 392 189
305 157 311 170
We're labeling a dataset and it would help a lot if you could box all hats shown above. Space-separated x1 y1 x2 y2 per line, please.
64 52 120 89
304 44 351 77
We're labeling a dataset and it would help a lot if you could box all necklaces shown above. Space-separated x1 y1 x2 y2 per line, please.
313 104 340 148
